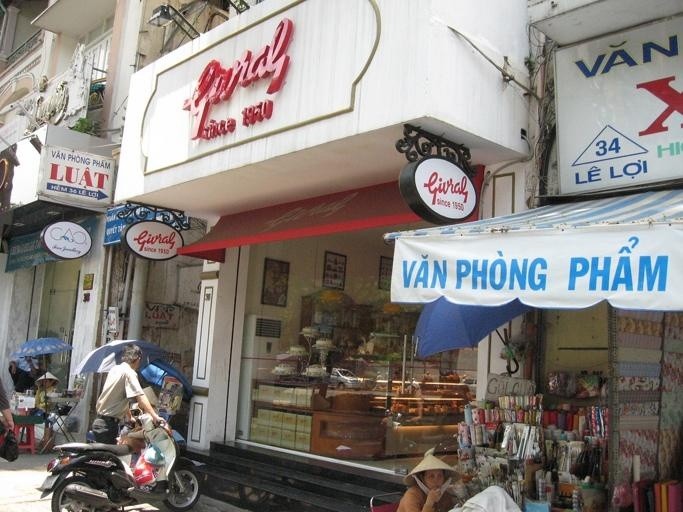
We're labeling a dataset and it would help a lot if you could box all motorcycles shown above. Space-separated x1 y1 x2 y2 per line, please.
36 408 208 511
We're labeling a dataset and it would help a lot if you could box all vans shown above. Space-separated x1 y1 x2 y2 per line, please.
327 368 361 391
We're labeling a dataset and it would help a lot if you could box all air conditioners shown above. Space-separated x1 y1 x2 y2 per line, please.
234 312 284 441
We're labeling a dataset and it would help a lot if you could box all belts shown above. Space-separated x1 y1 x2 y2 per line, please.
96 414 120 423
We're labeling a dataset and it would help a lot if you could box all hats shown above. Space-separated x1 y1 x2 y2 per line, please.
404 455 460 488
34 372 61 387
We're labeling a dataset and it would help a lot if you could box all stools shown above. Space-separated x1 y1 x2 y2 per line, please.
9 421 36 456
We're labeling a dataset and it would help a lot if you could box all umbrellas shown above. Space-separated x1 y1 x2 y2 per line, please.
11 337 73 425
142 358 192 402
74 340 170 374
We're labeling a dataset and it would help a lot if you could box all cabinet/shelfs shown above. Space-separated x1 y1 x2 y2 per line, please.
249 290 477 460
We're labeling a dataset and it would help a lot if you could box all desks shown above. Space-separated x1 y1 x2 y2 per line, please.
37 396 80 455
10 408 44 442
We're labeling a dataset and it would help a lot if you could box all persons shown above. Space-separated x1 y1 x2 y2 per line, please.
0 365 59 437
396 455 456 511
90 344 173 512
16 353 39 375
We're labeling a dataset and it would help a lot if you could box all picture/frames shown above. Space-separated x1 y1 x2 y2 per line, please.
260 257 290 308
320 249 347 291
377 254 393 291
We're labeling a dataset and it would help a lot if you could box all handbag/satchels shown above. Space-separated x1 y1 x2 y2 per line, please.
0 429 19 464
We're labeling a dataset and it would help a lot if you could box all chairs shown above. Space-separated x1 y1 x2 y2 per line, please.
368 491 404 511
